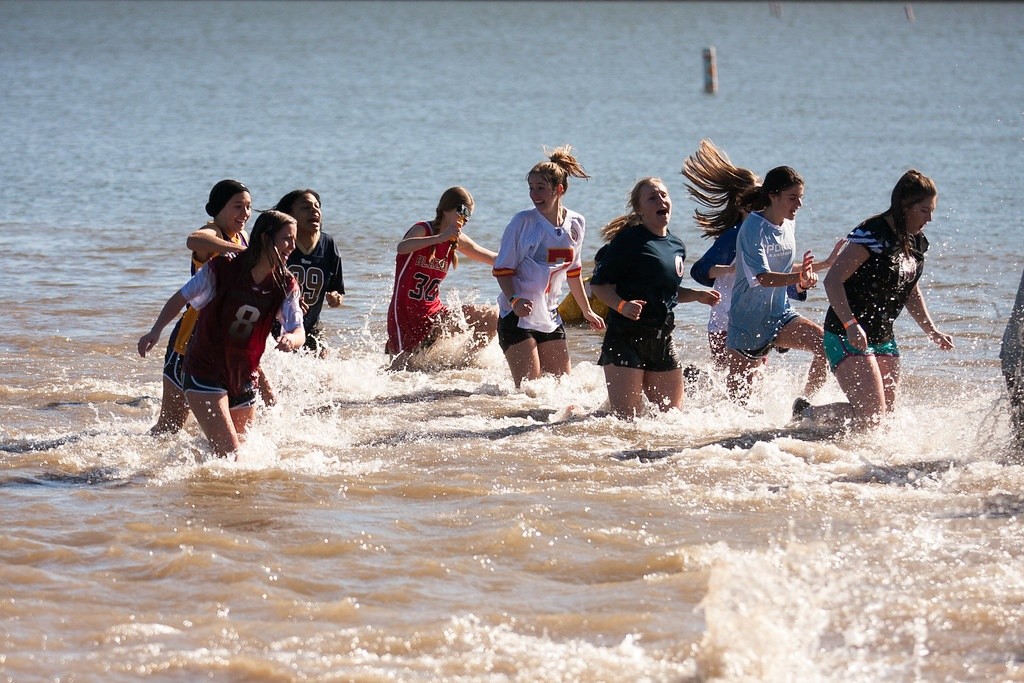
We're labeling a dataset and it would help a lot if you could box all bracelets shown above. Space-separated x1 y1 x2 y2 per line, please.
799 272 807 283
618 301 626 313
844 318 857 329
508 295 519 307
799 284 808 290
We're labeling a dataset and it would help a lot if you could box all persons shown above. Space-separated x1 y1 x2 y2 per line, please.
385 187 498 357
681 139 848 382
276 189 345 359
792 169 954 418
492 144 606 389
137 209 306 458
726 166 830 406
590 178 721 418
147 180 281 437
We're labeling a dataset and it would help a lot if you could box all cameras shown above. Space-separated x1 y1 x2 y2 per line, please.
456 204 469 223
